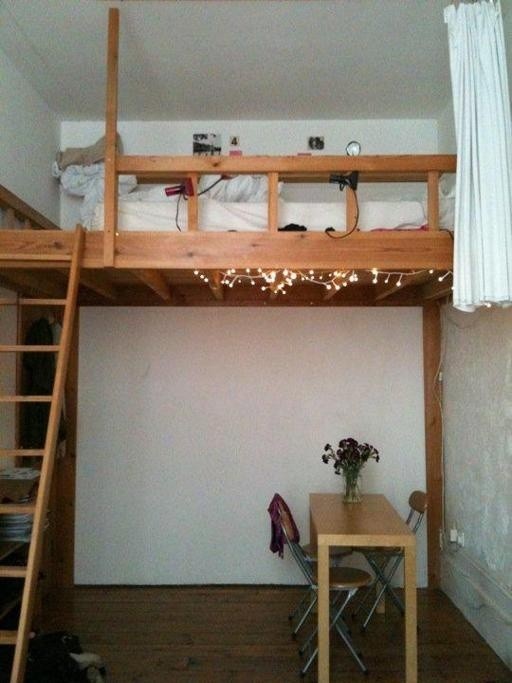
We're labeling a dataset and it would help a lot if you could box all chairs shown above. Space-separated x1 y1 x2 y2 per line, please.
352 491 427 631
269 494 372 675
289 540 354 638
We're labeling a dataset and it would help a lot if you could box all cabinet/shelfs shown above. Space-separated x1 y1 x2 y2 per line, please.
0 520 56 682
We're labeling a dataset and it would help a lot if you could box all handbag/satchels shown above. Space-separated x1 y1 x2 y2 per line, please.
25 630 89 682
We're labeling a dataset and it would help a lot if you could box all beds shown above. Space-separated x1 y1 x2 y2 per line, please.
0 153 457 682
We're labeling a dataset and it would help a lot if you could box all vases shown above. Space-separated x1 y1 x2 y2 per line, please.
340 471 364 505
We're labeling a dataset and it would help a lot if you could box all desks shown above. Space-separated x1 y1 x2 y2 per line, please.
308 494 421 682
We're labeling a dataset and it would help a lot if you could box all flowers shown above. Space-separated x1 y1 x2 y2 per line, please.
320 436 381 502
